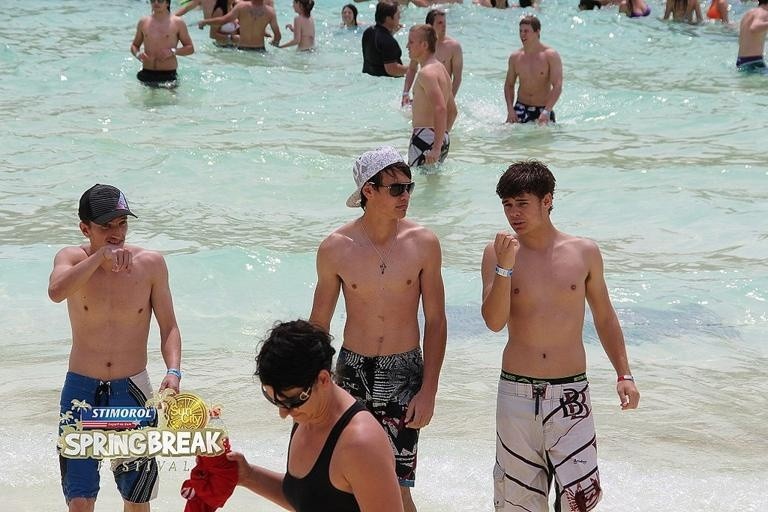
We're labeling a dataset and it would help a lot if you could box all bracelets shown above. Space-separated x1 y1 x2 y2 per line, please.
541 109 552 118
402 92 408 96
135 52 141 59
495 264 514 278
618 374 634 382
171 47 177 56
166 368 182 381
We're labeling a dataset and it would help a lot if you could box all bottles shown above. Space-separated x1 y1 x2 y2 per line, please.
201 410 228 458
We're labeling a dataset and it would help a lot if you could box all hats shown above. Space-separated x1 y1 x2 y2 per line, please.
78 183 139 226
345 144 407 210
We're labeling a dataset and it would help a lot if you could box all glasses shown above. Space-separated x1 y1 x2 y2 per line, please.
259 379 315 411
370 181 418 197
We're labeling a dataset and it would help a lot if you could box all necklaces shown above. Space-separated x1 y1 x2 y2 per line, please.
354 211 406 276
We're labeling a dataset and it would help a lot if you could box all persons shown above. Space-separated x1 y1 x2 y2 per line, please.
401 10 463 108
47 182 183 512
130 1 195 90
504 16 563 126
307 145 448 512
225 317 405 512
736 0 768 76
480 162 640 511
407 24 458 169
578 0 732 30
